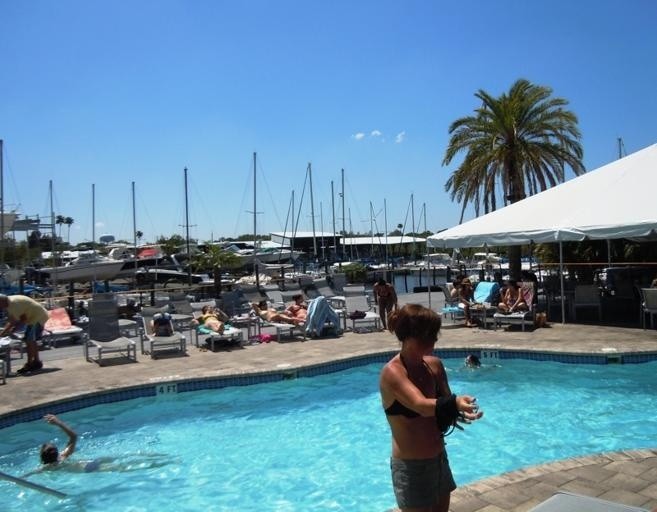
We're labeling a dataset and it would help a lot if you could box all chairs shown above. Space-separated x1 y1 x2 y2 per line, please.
437 274 657 333
0 273 381 384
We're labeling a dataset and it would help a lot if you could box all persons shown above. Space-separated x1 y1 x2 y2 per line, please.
465 354 481 368
190 305 225 336
21 414 181 478
252 300 307 327
284 294 309 326
373 279 398 331
451 269 538 328
1 294 49 373
379 303 484 511
650 278 657 288
